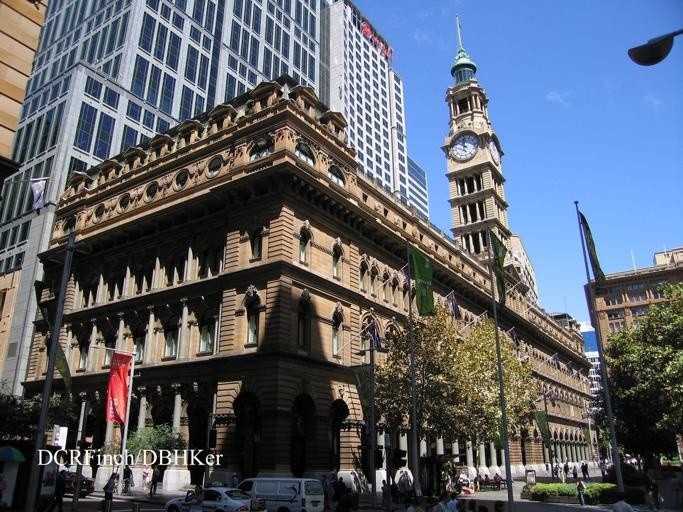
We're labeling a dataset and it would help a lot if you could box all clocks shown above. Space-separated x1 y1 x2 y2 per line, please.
449 131 479 162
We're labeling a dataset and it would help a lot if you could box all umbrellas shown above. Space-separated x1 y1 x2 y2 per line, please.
0 445 26 463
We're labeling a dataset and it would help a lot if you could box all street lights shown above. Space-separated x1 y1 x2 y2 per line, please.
360 349 389 509
628 29 683 66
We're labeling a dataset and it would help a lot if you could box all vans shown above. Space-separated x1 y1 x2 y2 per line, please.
236 478 325 512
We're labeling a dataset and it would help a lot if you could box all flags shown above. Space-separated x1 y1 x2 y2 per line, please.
547 389 558 407
507 329 520 347
32 180 45 211
402 264 416 302
365 321 380 347
447 292 462 322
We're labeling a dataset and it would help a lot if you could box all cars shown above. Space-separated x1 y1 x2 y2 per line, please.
165 487 252 512
65 472 94 497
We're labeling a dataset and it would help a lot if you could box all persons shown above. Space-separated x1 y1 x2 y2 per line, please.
44 470 67 512
149 463 160 496
612 491 634 512
142 463 149 490
551 457 591 484
381 470 507 512
576 477 585 505
124 465 133 492
230 472 238 488
645 471 661 511
598 461 607 476
671 473 682 505
103 473 117 511
185 484 204 512
321 473 359 510
